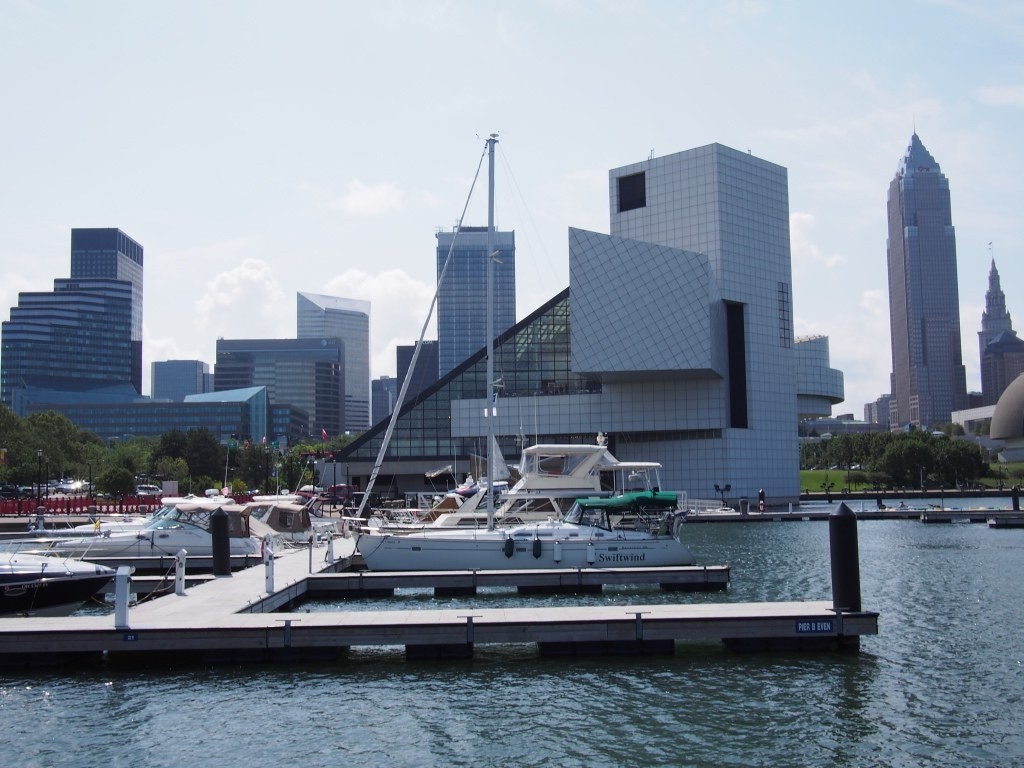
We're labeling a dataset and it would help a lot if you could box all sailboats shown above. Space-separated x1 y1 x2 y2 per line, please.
315 131 698 573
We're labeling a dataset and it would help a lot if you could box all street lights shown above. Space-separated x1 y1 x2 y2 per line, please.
306 460 318 494
224 433 235 498
37 448 43 514
86 462 92 498
264 445 270 495
46 458 49 503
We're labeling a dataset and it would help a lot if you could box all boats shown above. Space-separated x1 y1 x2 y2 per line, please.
0 532 118 618
0 440 690 574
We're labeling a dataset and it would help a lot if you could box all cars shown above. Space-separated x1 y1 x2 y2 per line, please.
0 477 96 498
243 489 260 496
136 485 163 497
